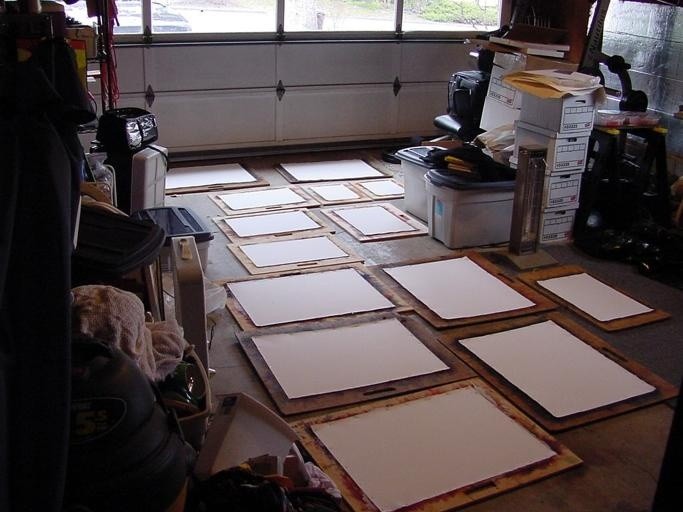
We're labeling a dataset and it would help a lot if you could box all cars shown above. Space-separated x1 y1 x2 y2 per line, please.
63 0 328 34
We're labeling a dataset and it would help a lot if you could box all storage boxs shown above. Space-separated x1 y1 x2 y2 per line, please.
87 140 227 449
433 35 605 136
394 123 605 253
196 392 301 479
